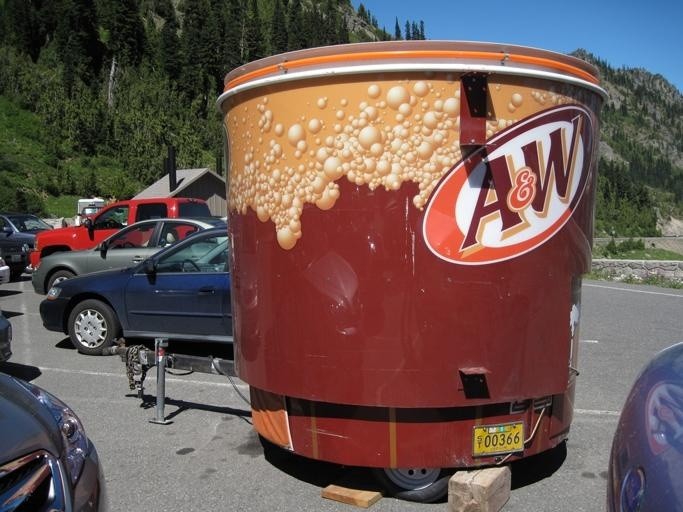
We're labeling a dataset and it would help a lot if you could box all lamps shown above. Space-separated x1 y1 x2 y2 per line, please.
163 230 179 247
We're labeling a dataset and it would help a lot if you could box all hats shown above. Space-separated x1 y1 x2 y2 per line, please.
0 199 237 365
1 371 101 512
